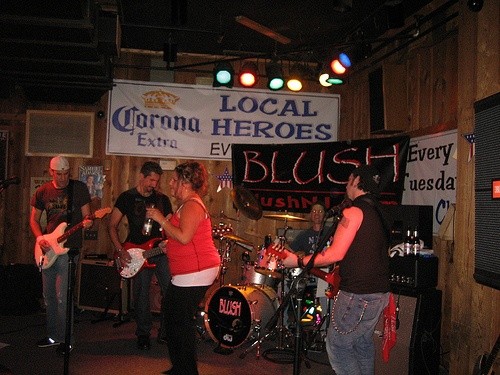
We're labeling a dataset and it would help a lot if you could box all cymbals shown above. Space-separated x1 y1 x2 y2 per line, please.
210 213 240 223
262 214 310 222
225 234 248 243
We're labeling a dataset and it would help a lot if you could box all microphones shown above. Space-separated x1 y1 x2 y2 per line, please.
325 198 352 217
0 177 21 185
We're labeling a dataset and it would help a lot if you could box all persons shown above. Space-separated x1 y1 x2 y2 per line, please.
146 162 220 375
30 156 93 354
282 165 392 375
277 202 335 334
107 161 175 351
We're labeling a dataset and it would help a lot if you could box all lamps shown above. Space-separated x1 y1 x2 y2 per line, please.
213 40 374 92
163 32 177 62
467 0 484 12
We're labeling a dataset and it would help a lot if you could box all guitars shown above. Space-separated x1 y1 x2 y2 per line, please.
113 224 235 279
35 207 112 270
265 242 341 298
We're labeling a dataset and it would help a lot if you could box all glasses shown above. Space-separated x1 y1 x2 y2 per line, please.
311 210 324 214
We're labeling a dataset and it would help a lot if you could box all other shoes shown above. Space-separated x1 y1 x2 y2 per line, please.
56 343 73 355
36 337 61 347
158 336 168 346
137 336 151 350
162 367 199 375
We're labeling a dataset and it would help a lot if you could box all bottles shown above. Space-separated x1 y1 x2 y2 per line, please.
413 226 420 256
142 203 154 235
404 226 412 256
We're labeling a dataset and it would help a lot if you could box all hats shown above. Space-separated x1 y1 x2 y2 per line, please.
50 156 70 171
352 166 383 192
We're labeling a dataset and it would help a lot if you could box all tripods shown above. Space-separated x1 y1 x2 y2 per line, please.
240 237 311 369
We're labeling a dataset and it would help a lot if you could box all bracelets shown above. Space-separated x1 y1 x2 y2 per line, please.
160 218 169 227
36 236 45 243
117 248 126 254
297 256 305 268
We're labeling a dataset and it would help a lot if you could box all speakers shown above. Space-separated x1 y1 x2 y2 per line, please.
77 259 129 315
371 284 442 375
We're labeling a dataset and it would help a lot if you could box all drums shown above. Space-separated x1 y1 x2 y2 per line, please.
286 267 318 286
204 283 282 348
241 246 277 286
255 245 291 278
